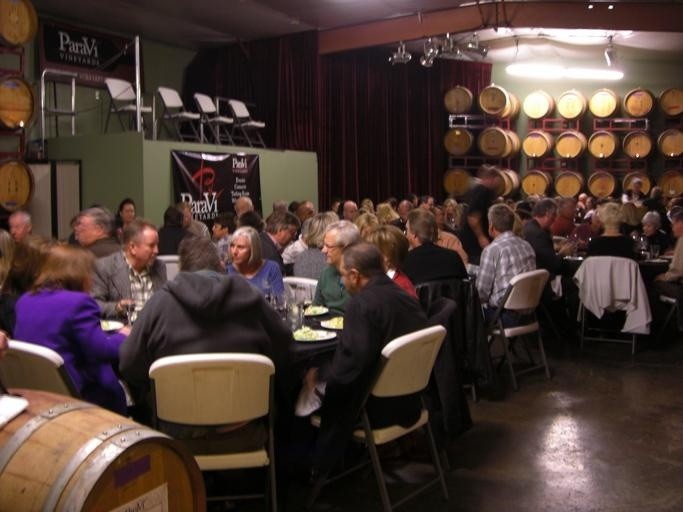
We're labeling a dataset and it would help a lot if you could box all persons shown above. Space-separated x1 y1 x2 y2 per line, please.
476 204 537 329
550 197 576 238
74 208 121 259
521 199 574 277
118 236 303 456
294 211 339 281
312 220 360 316
158 206 195 255
654 212 683 283
0 329 9 401
361 225 418 302
0 211 58 331
435 229 468 269
211 217 230 247
404 208 467 288
225 226 284 304
259 211 302 275
587 202 637 264
234 179 462 225
116 197 136 236
87 220 168 327
303 240 426 430
178 202 211 240
463 169 502 265
13 245 133 415
528 179 683 210
641 211 668 257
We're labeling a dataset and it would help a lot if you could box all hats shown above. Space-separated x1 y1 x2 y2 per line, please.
376 202 400 224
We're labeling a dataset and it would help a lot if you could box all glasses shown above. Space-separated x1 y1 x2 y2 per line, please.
323 241 343 248
287 227 297 238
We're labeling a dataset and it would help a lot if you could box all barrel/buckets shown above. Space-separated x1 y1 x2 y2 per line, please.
556 90 587 121
554 170 585 198
0 156 34 213
522 130 555 160
506 129 520 156
0 389 206 512
478 127 512 158
659 87 683 117
479 84 512 119
588 131 619 162
589 88 619 118
521 170 553 197
557 131 587 158
522 91 554 119
657 129 683 158
622 131 653 160
0 72 36 136
658 171 683 199
622 172 651 198
588 170 618 200
444 166 473 197
0 0 36 47
444 130 474 155
442 86 470 114
623 89 656 117
506 92 520 121
501 168 519 198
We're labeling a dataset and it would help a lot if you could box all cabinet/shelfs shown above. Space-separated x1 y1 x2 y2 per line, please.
0 45 25 220
26 157 82 244
592 117 649 172
447 114 511 170
527 118 580 173
662 114 683 173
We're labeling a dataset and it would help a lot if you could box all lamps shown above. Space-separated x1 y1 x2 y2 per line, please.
443 34 460 55
506 33 623 80
389 41 411 64
461 32 489 59
420 38 438 66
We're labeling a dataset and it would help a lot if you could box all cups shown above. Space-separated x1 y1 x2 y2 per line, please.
570 231 580 245
286 300 303 327
295 283 312 303
640 233 647 250
119 287 136 324
650 245 660 259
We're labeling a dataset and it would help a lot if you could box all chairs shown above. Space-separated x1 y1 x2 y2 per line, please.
157 87 201 143
414 274 477 405
227 100 266 148
194 93 235 145
487 268 552 392
148 352 276 511
305 323 449 512
105 77 152 137
0 338 81 399
281 276 318 306
580 257 640 356
653 279 683 332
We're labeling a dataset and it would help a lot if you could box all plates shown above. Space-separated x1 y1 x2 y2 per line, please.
320 320 345 330
294 328 337 342
304 307 328 316
